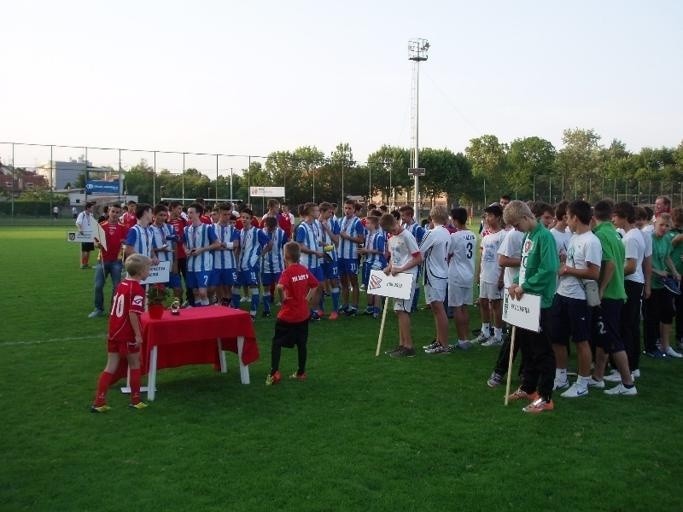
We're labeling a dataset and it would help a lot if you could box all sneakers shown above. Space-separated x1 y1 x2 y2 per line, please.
289 373 306 381
263 312 272 320
129 402 147 409
240 297 252 302
487 371 503 388
422 338 441 349
603 384 638 396
657 274 680 295
339 305 350 314
182 301 189 307
265 371 280 386
456 339 472 350
425 345 456 354
560 382 589 397
603 370 635 382
588 375 604 388
328 311 339 319
522 398 554 413
504 385 539 400
91 405 112 413
552 378 570 391
472 328 493 335
250 315 256 322
362 310 373 316
642 349 667 359
480 336 504 347
672 344 683 353
88 310 103 318
350 310 358 317
390 347 416 358
385 345 403 355
610 369 640 377
470 331 491 343
311 311 320 321
372 312 379 318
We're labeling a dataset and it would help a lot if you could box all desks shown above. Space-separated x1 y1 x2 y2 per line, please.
126 306 250 402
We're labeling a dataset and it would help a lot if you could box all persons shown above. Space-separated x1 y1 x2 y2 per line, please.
90 255 153 413
503 200 561 413
67 194 683 367
487 203 555 388
589 201 638 396
53 205 59 220
554 202 603 401
266 240 319 386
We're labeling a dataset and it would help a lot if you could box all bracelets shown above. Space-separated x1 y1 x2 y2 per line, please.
564 267 568 275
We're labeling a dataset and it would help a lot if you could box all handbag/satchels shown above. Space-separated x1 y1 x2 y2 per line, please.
581 279 602 307
